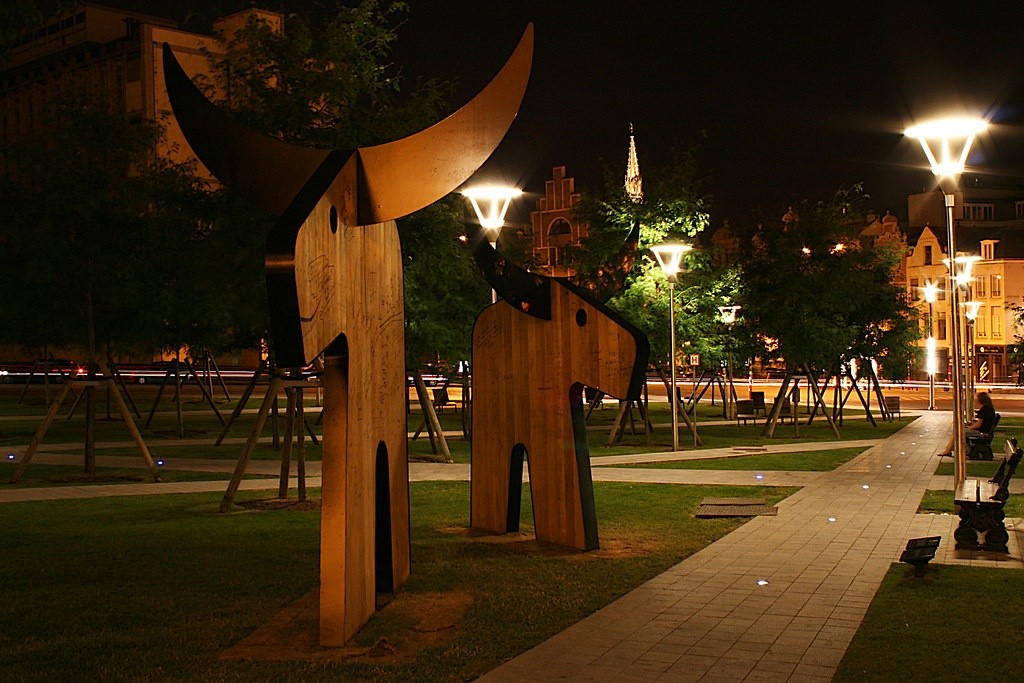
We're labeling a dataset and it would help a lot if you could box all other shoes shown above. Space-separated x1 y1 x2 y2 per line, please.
937 452 952 456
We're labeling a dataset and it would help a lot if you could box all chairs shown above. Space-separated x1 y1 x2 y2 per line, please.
750 391 767 416
885 396 901 421
736 399 757 427
774 397 793 424
584 387 604 411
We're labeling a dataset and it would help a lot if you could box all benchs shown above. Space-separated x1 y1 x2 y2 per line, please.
966 413 1002 460
954 437 1024 553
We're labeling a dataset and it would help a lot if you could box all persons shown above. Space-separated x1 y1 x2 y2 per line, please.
937 392 995 456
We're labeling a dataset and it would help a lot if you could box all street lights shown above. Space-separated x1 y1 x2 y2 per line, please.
924 286 937 411
648 241 695 453
903 112 988 512
941 253 983 427
717 304 742 420
459 179 524 305
